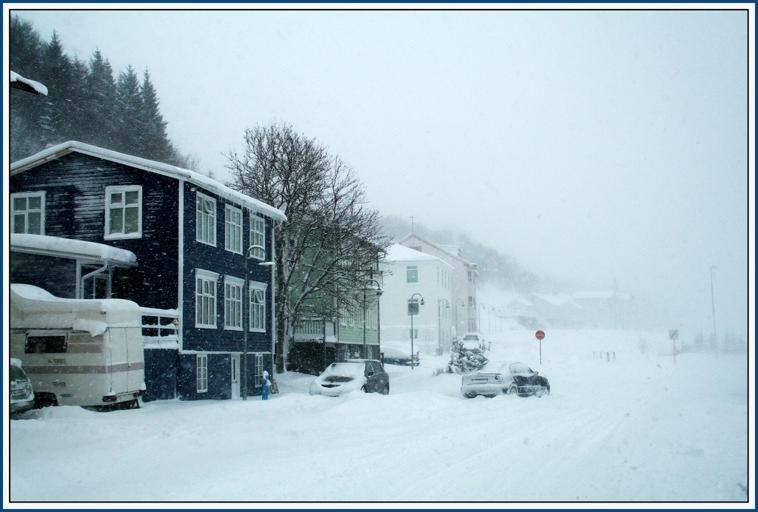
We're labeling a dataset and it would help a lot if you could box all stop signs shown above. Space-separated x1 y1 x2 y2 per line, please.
536 330 545 340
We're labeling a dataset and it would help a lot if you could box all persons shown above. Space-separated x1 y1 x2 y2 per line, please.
262 370 271 400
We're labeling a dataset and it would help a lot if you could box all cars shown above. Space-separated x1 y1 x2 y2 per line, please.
10 358 36 414
309 358 389 397
462 359 550 398
381 342 420 366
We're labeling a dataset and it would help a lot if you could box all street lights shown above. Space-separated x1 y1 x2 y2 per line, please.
439 297 494 356
411 292 424 369
241 245 276 398
363 279 383 359
